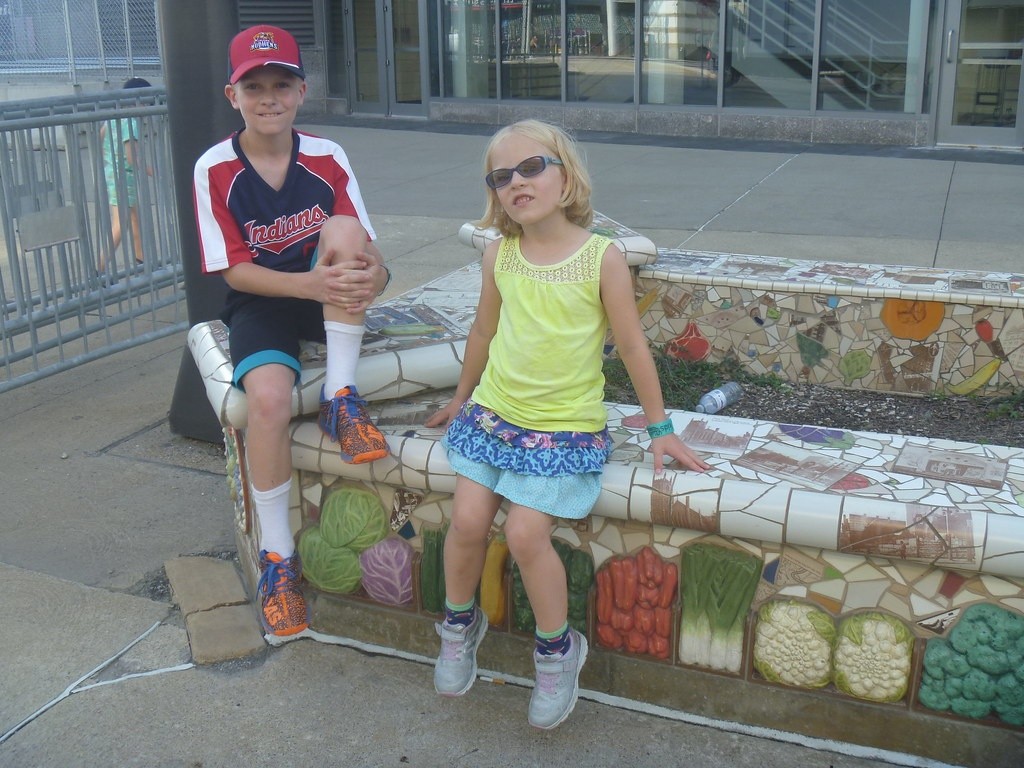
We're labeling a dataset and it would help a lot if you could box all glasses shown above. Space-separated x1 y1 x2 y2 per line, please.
484 154 563 189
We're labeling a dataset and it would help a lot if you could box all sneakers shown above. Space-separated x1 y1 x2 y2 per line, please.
256 548 311 636
434 603 490 697
317 383 392 464
527 626 589 730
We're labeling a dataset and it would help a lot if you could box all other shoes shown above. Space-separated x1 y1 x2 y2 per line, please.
94 270 120 288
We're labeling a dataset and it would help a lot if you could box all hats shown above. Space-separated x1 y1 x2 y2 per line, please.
227 25 306 89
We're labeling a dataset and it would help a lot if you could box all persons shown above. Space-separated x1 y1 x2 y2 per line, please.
86 76 162 290
421 117 710 738
187 23 390 646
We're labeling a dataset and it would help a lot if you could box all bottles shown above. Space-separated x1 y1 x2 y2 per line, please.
696 381 742 414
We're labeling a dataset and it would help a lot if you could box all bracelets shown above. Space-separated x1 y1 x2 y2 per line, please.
646 420 676 438
379 266 393 297
